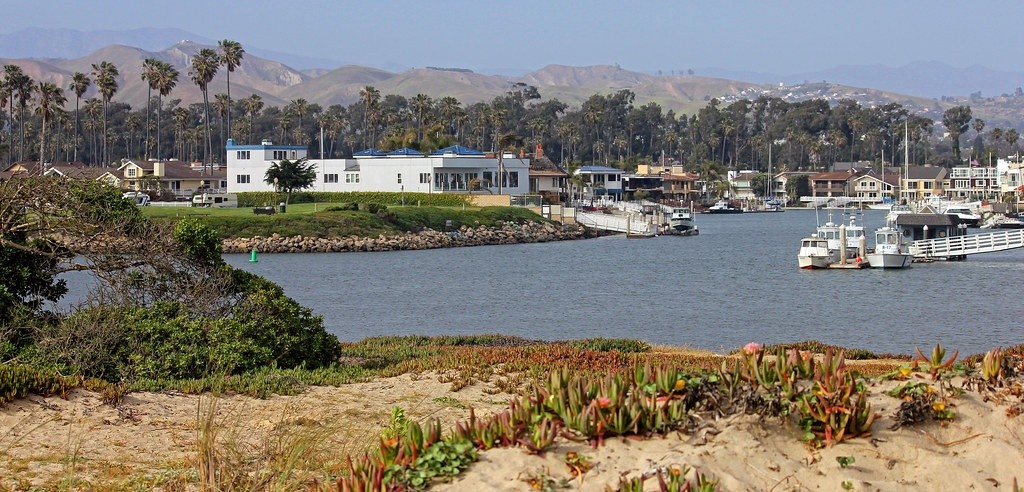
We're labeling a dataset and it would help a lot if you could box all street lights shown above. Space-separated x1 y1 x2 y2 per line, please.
273 177 278 213
200 180 205 207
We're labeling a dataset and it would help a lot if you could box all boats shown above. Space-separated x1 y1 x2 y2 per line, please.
865 222 915 268
121 192 151 207
798 180 870 270
890 120 1024 228
708 199 744 215
668 200 699 236
